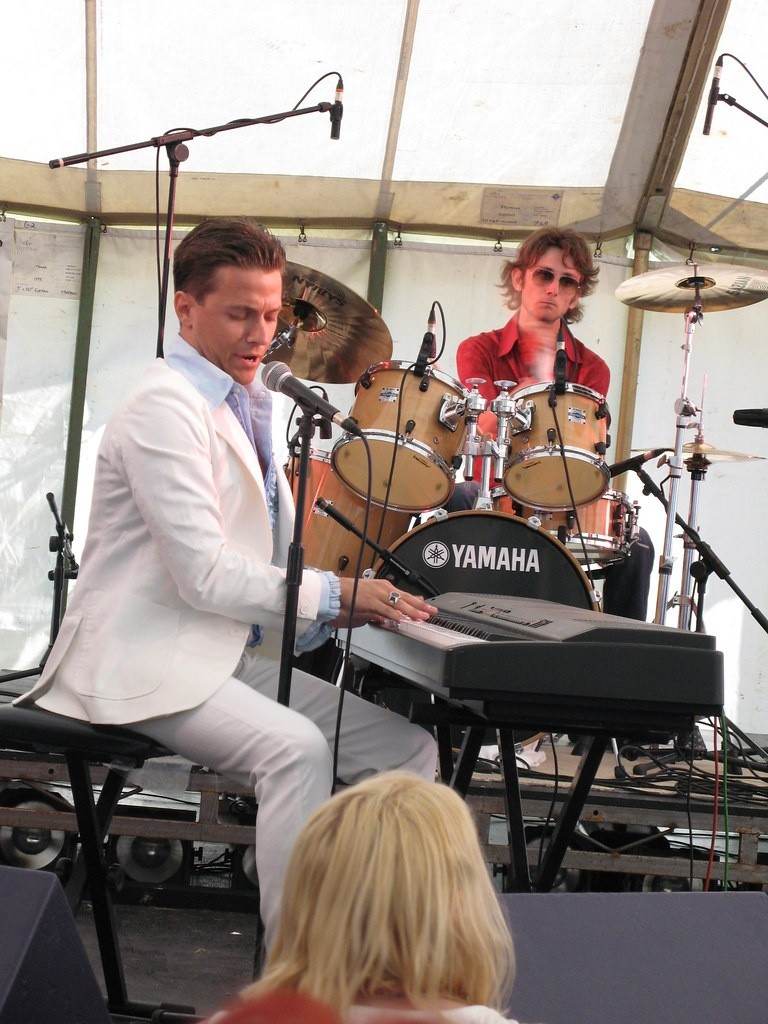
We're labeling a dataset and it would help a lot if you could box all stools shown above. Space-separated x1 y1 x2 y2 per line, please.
0 689 196 1020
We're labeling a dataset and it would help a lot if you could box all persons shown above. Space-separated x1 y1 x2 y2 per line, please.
197 771 522 1024
442 220 655 770
11 217 443 976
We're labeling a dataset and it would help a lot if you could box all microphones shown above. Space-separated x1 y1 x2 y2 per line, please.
261 361 363 436
733 408 768 428
554 328 567 377
608 448 666 478
702 56 723 135
427 310 436 358
320 392 332 439
330 81 343 140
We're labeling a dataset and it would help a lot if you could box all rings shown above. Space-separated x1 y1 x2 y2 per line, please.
388 593 400 605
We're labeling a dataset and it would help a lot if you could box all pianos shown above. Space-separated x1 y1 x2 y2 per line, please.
331 590 724 891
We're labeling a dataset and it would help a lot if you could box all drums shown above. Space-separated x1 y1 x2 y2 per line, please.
489 484 634 574
500 380 614 512
329 359 469 514
367 508 603 755
283 444 415 579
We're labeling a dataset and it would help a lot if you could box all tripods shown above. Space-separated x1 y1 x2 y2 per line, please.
623 468 768 778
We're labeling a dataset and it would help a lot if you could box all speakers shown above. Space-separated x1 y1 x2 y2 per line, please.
495 890 768 1024
0 865 115 1024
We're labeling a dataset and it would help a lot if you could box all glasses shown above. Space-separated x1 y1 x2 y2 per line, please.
529 267 580 294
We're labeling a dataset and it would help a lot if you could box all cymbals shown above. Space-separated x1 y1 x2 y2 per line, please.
260 260 394 385
629 441 768 462
613 262 768 315
